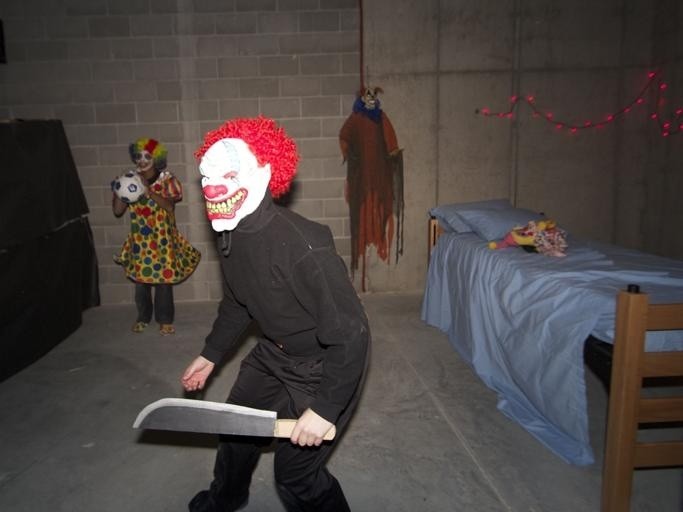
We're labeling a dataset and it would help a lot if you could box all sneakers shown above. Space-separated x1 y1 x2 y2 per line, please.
159 323 176 337
187 489 251 512
131 320 148 333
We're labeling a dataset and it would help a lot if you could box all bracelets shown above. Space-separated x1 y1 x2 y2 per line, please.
147 191 154 200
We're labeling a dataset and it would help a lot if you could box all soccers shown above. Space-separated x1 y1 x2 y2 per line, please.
112 172 145 204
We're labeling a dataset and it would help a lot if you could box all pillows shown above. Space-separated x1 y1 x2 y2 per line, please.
454 206 573 241
429 198 513 232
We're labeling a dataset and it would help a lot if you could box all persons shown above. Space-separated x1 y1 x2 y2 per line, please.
111 137 200 335
177 112 369 511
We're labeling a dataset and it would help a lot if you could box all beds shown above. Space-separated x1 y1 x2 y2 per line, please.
418 206 683 512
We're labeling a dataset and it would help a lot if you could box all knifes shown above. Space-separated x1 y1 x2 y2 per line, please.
131 397 338 441
